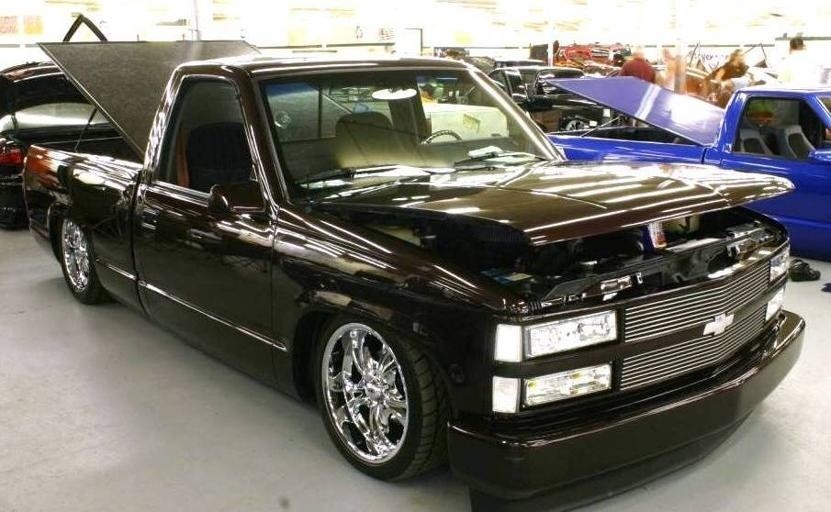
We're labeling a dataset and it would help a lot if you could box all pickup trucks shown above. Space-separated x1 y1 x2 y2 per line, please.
17 35 812 510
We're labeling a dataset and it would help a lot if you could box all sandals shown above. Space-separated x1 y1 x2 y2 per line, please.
789 258 821 281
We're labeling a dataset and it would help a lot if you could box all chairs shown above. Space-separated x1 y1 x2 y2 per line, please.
186 123 250 192
777 123 816 160
333 113 394 172
739 129 774 157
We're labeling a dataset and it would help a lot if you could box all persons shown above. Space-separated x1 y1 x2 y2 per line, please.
715 50 748 80
618 48 657 127
